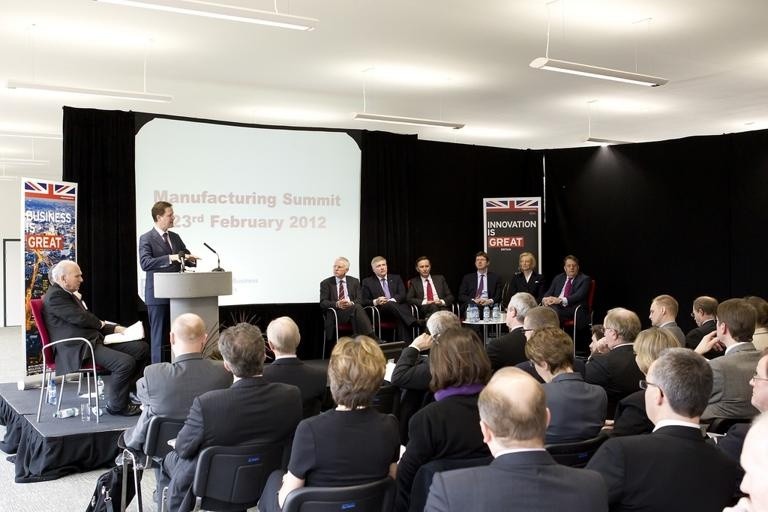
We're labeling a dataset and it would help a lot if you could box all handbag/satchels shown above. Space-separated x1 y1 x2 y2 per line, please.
85 465 143 512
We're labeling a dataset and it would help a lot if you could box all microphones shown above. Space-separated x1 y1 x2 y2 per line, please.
203 242 225 272
178 250 185 273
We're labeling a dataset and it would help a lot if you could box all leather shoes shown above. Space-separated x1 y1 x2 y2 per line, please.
129 392 142 405
106 404 142 416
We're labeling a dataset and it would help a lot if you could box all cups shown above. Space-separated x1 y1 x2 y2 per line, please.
80 403 90 421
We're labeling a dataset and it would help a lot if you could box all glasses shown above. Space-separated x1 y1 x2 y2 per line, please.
640 380 664 397
713 319 720 323
521 328 533 334
752 372 767 382
691 310 699 317
602 328 611 332
430 332 440 344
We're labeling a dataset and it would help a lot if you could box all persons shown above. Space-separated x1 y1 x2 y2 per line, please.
139 201 199 366
458 251 505 337
117 292 768 512
406 256 455 335
540 255 590 330
359 256 426 350
503 252 547 314
42 260 151 416
320 256 388 344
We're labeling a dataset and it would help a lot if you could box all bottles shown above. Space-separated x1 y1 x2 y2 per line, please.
53 408 78 419
473 305 480 322
469 305 474 321
466 304 471 321
98 376 104 398
48 380 57 405
92 407 103 416
483 304 490 320
492 303 500 321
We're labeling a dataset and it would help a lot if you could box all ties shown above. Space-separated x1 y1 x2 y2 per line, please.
381 279 391 299
339 280 345 300
426 279 433 301
162 233 172 254
564 278 573 297
478 275 485 296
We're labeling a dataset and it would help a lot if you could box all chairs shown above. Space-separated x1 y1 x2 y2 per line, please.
30 299 104 422
281 476 396 512
121 414 186 512
704 418 752 441
320 279 596 361
321 384 405 416
190 438 288 512
546 433 613 469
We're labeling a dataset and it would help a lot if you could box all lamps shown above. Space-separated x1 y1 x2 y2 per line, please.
91 1 319 32
5 24 174 103
585 104 634 144
353 71 464 131
529 0 669 87
